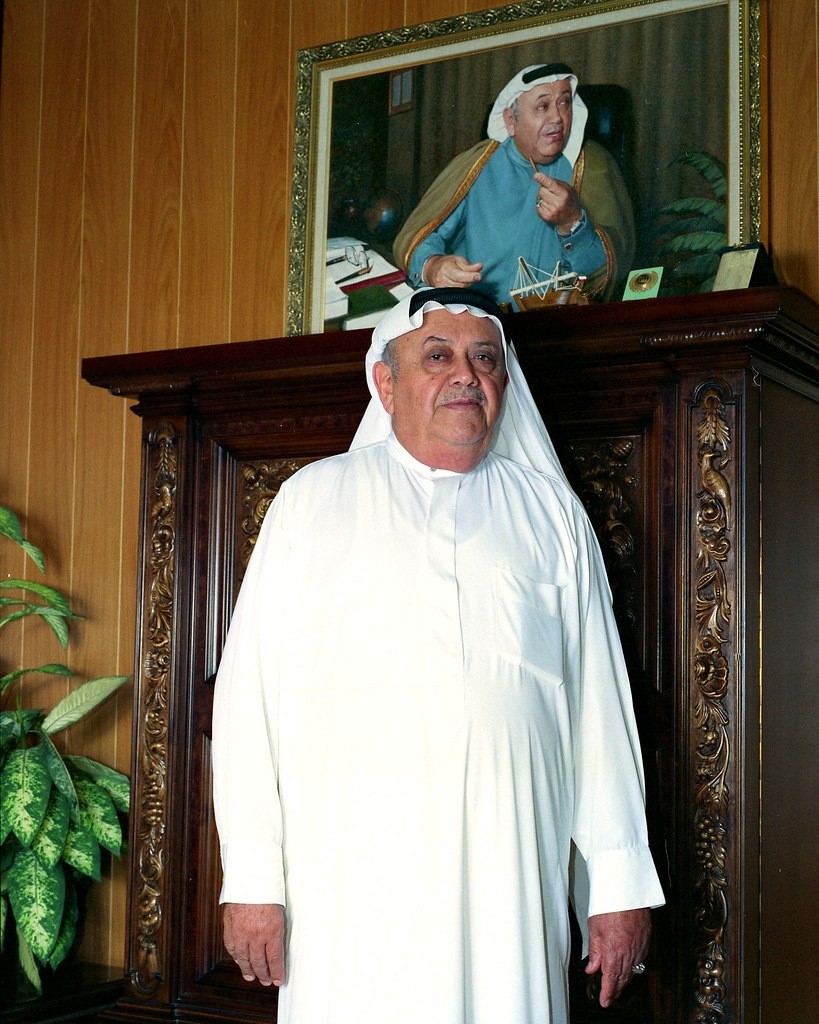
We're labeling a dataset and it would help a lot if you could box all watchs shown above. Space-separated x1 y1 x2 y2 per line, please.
557 213 585 237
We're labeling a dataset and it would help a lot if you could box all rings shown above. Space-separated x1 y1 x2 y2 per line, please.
631 962 646 974
536 199 542 207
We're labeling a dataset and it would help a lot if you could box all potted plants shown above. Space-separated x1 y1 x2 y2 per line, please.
0 503 140 997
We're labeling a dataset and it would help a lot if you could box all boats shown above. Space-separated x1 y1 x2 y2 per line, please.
509 254 586 311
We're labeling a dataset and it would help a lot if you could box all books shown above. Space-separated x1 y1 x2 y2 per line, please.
325 237 414 331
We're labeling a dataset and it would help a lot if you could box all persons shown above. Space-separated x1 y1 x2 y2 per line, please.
392 63 635 314
213 288 667 1024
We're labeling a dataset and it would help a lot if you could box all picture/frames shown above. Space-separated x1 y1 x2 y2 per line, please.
282 0 772 337
